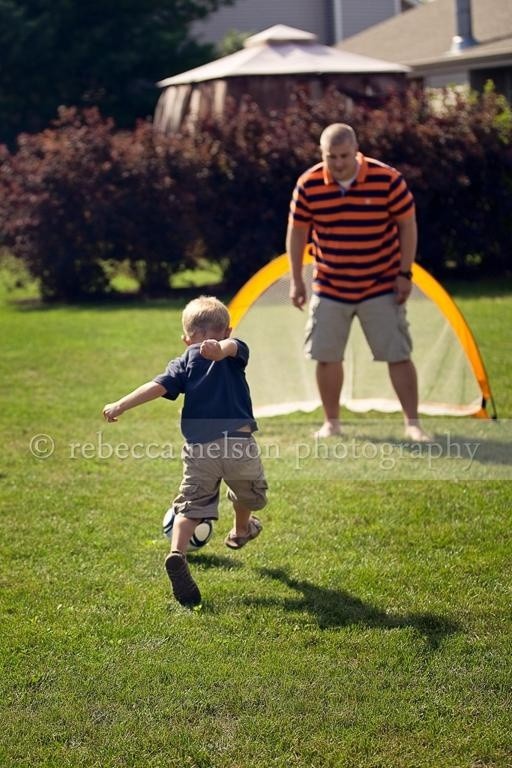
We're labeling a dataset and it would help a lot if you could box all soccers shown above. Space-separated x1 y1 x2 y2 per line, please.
163 507 212 552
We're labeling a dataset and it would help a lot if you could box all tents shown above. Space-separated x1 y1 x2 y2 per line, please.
225 243 499 421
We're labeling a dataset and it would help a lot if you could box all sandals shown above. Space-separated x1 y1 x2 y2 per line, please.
164 549 200 602
223 514 262 549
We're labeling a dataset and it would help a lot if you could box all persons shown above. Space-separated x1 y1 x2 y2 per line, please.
101 295 267 608
287 117 434 447
